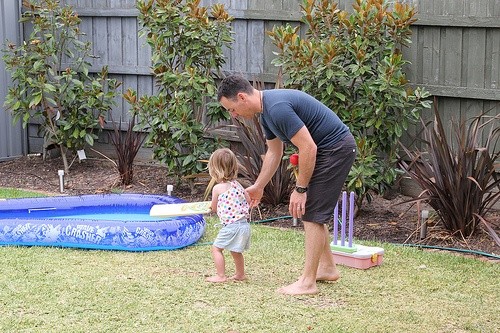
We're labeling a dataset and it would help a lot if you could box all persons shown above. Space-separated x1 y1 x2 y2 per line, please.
206 147 251 285
217 74 356 295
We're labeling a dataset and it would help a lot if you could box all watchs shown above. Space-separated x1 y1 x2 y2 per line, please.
295 186 309 193
